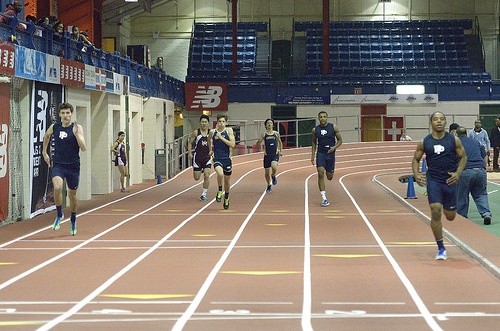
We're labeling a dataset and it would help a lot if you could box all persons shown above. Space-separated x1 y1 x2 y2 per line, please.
188 115 211 201
311 111 342 206
412 111 467 260
253 118 283 193
43 103 86 235
469 118 500 170
112 131 128 192
449 123 492 225
208 114 235 209
400 130 412 141
0 0 24 41
26 14 96 62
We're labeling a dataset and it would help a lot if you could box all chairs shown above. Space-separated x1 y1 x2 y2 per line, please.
189 19 268 78
292 17 492 86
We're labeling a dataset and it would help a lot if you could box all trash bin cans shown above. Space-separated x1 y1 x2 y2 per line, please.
154 148 172 178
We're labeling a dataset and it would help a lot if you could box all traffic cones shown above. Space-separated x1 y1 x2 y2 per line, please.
421 158 429 173
404 175 419 201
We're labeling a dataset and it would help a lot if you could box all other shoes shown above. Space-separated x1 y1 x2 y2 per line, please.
266 185 272 193
271 174 277 185
223 196 230 210
52 212 65 232
69 219 78 236
216 191 222 202
484 216 491 225
321 200 330 207
200 195 208 202
435 248 448 261
